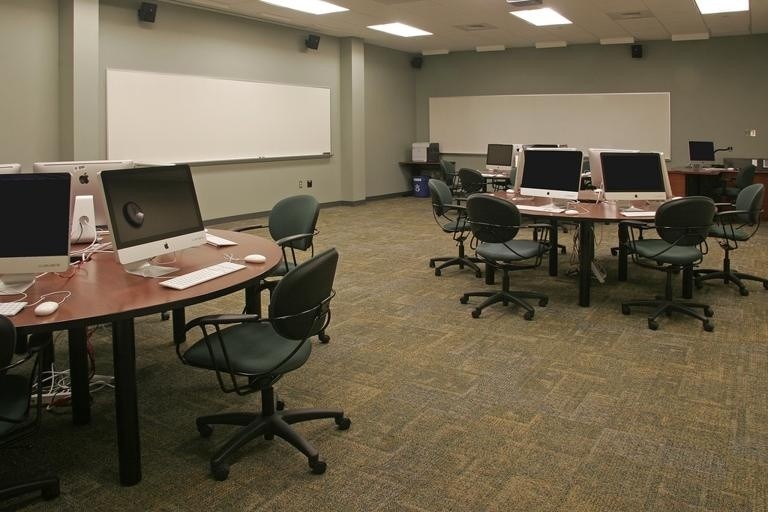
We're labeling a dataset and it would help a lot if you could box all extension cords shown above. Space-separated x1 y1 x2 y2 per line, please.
30 392 72 406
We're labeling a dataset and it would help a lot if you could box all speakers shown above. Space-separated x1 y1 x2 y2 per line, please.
309 35 320 49
414 57 422 68
632 45 642 58
142 2 156 22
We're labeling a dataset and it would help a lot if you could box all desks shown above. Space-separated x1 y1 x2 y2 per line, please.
0 230 285 489
668 169 768 223
492 190 666 306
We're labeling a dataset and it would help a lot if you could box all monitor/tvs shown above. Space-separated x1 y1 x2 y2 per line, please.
0 160 208 295
482 144 672 217
689 141 715 170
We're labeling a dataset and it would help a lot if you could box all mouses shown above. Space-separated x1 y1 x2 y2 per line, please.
244 254 266 263
34 301 59 316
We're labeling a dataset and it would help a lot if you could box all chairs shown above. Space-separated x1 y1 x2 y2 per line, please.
232 193 332 344
173 246 354 481
459 194 552 320
0 312 63 505
527 220 577 254
694 183 768 297
440 158 517 199
621 196 714 332
611 223 664 265
428 179 485 278
714 164 755 203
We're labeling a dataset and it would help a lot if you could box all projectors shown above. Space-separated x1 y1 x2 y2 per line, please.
506 0 542 7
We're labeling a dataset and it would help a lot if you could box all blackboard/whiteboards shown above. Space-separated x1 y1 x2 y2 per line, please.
106 69 332 167
429 91 671 161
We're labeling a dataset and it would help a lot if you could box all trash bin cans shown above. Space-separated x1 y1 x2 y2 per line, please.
412 176 429 197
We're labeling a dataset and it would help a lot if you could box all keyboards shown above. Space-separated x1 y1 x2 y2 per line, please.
206 232 238 248
0 301 28 316
158 262 246 291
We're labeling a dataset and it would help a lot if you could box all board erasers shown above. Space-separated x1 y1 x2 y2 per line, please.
323 152 330 154
